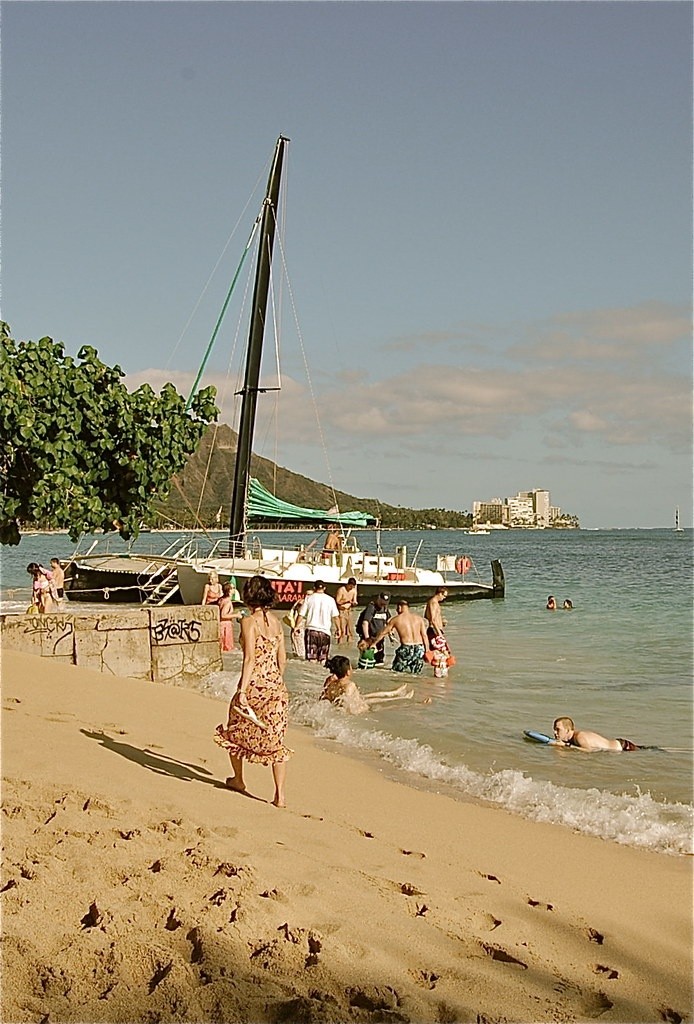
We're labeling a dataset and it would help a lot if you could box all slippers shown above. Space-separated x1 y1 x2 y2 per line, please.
233 704 267 731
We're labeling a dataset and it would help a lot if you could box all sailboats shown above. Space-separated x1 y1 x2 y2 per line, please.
671 506 686 531
54 132 508 610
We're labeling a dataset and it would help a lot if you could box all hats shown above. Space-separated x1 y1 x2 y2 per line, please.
379 591 391 606
314 580 326 590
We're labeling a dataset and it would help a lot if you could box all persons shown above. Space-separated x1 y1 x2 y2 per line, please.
425 587 454 679
563 599 573 609
320 656 430 708
336 577 359 645
291 581 341 663
201 571 224 605
546 595 556 609
27 558 64 613
553 716 662 753
217 581 242 651
357 590 430 675
214 576 285 808
322 530 339 558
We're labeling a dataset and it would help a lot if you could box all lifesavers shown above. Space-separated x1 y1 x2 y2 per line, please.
456 558 470 574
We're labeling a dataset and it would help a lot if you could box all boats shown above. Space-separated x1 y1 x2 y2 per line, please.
462 529 491 535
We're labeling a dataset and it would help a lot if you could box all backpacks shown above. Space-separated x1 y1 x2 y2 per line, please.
356 603 377 635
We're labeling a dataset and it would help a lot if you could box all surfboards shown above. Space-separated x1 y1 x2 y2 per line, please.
523 729 550 743
